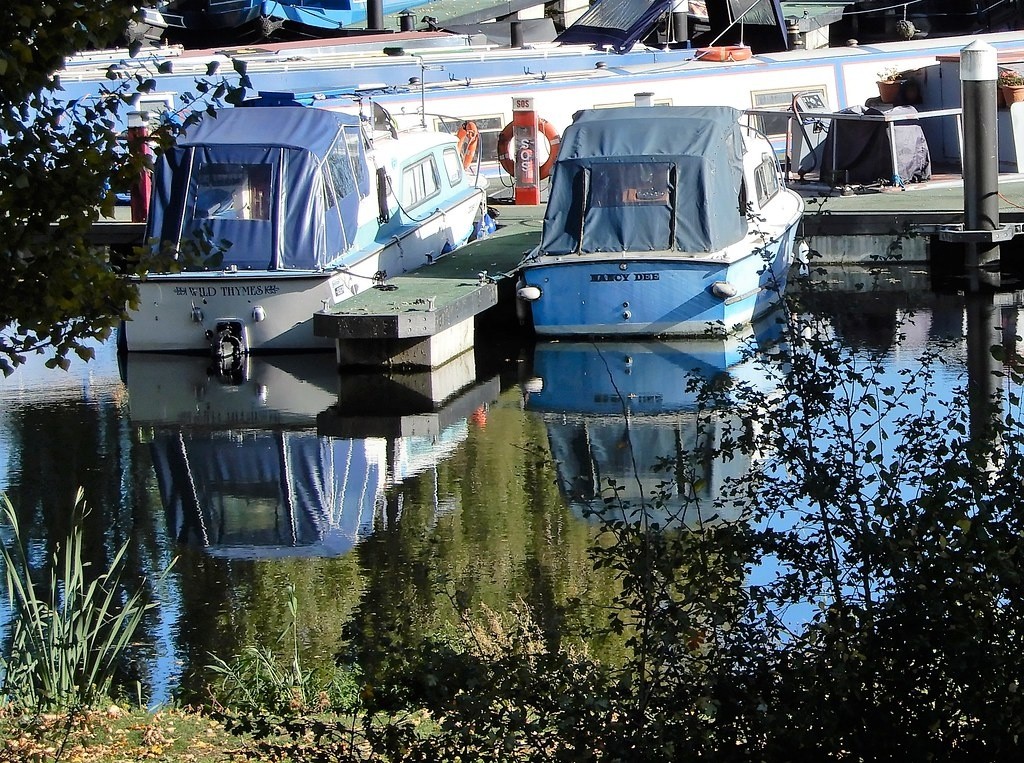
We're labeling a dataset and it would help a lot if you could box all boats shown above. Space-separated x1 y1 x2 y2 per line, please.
523 294 793 507
125 350 467 562
516 102 813 339
110 83 491 352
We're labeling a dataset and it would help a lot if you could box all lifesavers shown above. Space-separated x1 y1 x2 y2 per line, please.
455 121 479 170
496 116 562 182
696 44 753 62
